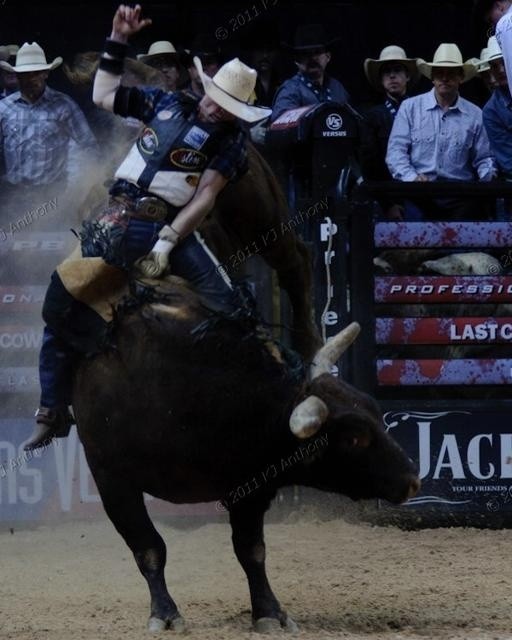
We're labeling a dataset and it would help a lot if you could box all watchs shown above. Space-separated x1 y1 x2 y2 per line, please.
157 224 183 245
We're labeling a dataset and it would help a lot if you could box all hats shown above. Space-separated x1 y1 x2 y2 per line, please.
0 42 63 74
181 37 229 62
364 45 418 86
416 35 504 84
137 40 180 63
193 55 273 123
280 26 338 56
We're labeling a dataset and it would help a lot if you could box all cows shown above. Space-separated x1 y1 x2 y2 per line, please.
61 49 423 640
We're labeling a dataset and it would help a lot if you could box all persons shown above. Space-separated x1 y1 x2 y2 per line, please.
18 3 273 451
0 1 511 223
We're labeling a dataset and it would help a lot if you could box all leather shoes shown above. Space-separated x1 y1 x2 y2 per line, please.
22 406 72 450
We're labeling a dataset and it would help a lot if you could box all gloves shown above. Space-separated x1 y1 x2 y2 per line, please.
142 225 183 278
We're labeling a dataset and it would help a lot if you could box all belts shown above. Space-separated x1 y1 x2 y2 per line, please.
130 196 174 223
